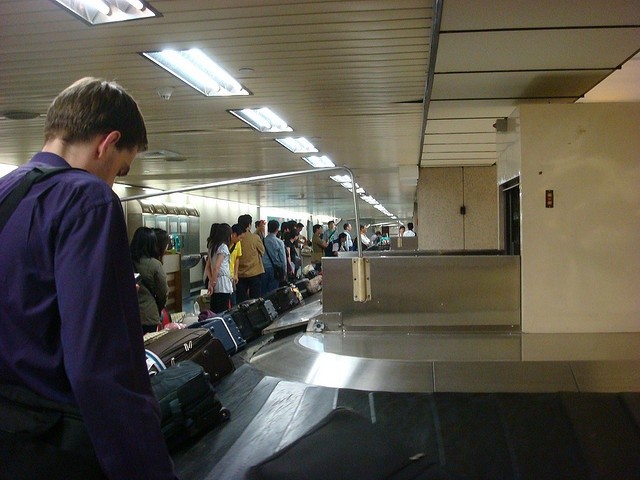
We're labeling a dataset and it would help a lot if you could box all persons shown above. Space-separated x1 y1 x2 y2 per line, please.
404 222 416 236
397 226 406 235
323 220 338 255
127 226 171 333
331 233 348 257
255 220 267 244
311 224 330 271
373 231 389 249
205 223 233 313
262 220 287 295
342 223 354 251
281 220 305 279
235 213 265 297
0 76 163 479
229 222 246 306
354 224 370 250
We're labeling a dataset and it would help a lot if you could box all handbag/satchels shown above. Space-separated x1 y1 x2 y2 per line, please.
309 275 323 293
295 250 301 270
262 238 284 281
311 236 325 263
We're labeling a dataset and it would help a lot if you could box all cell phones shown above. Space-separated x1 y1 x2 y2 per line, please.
133 272 142 287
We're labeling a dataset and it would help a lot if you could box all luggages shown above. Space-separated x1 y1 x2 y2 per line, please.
223 297 282 343
262 286 300 312
294 278 311 298
149 359 230 455
143 326 236 383
246 407 438 480
186 313 249 356
